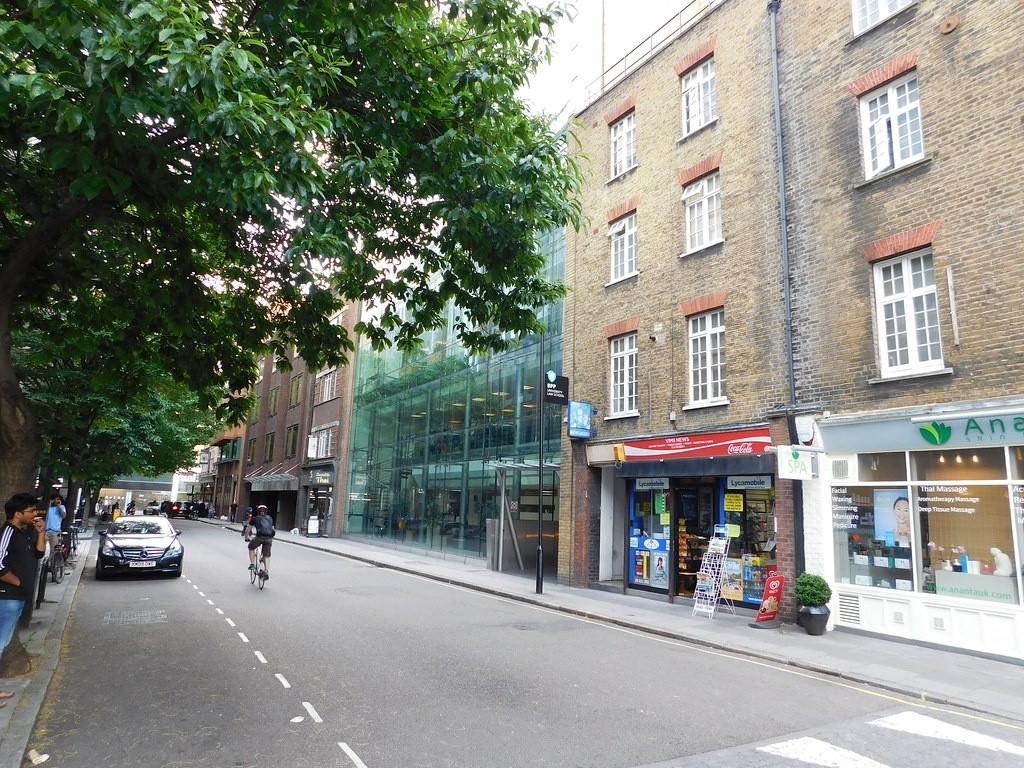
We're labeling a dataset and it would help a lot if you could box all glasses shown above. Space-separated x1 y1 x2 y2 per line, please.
22 508 39 514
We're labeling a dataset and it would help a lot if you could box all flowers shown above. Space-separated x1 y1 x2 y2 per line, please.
927 541 966 559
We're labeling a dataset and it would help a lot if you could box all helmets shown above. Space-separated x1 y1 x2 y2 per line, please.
257 505 268 514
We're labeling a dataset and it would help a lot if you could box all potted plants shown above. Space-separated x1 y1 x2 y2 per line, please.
794 571 833 636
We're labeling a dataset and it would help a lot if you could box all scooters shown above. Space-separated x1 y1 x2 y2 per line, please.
127 504 136 516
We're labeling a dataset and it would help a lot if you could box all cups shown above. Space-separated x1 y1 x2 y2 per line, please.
967 561 980 574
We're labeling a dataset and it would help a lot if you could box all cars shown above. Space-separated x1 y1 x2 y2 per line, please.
96 515 185 580
162 499 209 521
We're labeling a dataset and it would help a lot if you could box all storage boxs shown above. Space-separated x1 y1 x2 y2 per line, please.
751 556 768 567
752 566 769 581
967 561 980 574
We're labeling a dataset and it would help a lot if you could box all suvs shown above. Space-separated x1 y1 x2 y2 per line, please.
143 501 164 516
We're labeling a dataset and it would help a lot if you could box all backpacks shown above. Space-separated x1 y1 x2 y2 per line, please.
257 515 275 536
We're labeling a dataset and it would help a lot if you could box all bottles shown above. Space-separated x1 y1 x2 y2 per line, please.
858 543 862 555
873 541 882 550
899 532 913 547
953 559 962 572
875 546 883 556
945 560 952 571
861 545 867 555
961 550 968 573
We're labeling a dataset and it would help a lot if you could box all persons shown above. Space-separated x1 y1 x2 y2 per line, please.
126 500 135 513
186 500 215 519
95 497 120 521
0 493 46 708
45 494 66 559
242 507 253 536
890 497 911 541
244 505 273 579
656 557 663 570
230 501 238 522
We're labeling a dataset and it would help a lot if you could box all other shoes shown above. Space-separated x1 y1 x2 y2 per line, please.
0 701 7 708
249 532 252 536
264 573 269 580
249 564 255 570
242 532 244 536
0 688 14 699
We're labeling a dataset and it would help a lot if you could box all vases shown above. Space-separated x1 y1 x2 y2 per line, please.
941 562 952 570
944 560 953 571
953 558 962 572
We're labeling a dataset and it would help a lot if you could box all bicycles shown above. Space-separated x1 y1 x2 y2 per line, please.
245 539 268 590
66 522 82 556
46 528 73 584
